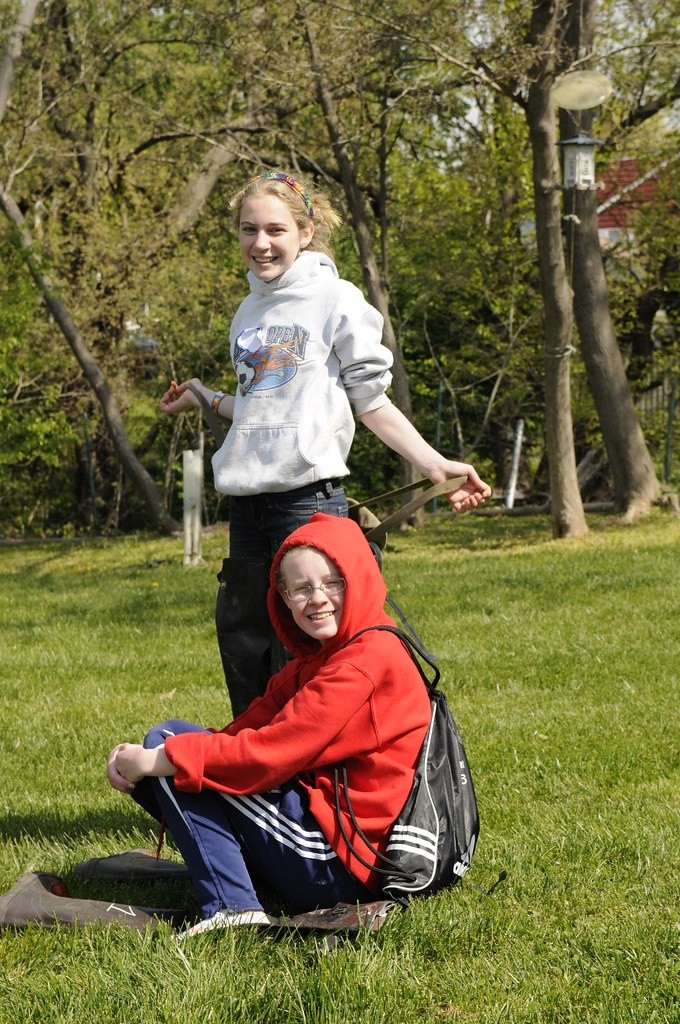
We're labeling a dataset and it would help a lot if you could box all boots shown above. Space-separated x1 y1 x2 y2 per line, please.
0 870 176 945
81 848 194 882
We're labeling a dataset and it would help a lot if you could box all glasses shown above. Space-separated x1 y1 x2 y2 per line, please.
283 578 347 602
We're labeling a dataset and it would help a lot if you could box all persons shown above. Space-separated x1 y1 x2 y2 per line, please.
105 514 434 942
159 172 492 724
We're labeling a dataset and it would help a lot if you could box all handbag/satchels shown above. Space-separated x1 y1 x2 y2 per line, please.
257 900 395 972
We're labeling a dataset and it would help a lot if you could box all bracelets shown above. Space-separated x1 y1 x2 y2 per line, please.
211 391 228 416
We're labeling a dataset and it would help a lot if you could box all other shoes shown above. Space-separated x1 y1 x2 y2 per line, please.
168 911 271 944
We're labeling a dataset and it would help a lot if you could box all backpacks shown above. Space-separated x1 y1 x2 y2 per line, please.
295 597 480 906
168 375 470 574
